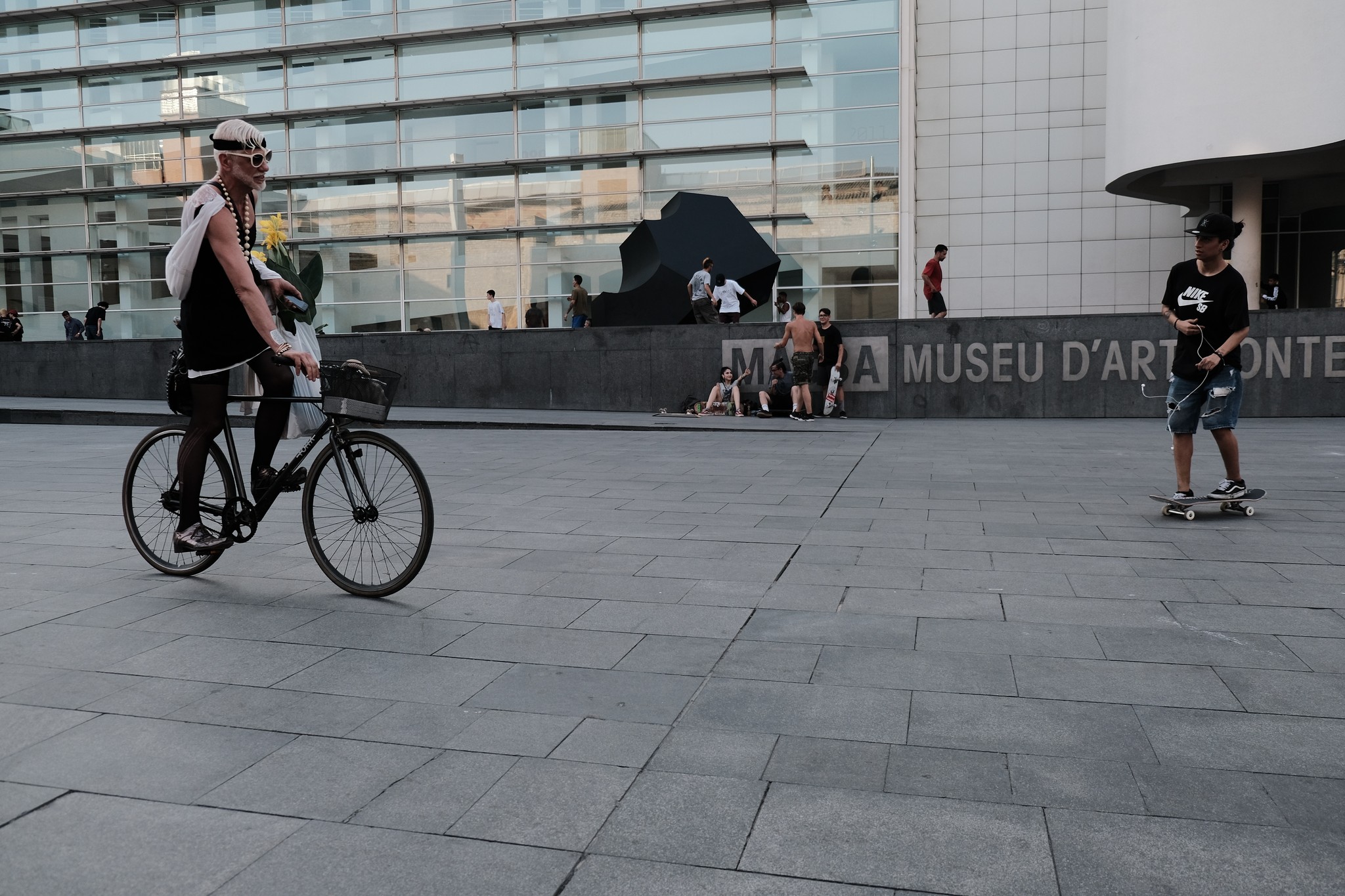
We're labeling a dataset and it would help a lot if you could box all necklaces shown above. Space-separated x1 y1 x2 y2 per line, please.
217 170 251 263
725 382 731 390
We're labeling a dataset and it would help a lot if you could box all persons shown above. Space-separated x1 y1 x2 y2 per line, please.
1259 273 1285 310
564 275 588 328
525 299 547 328
811 308 847 418
921 244 948 319
584 319 593 327
756 358 805 419
83 301 109 340
1160 212 1250 510
773 292 792 323
711 274 757 323
774 302 824 422
486 290 507 331
687 258 720 325
698 366 751 417
0 308 24 342
62 310 85 340
163 119 321 555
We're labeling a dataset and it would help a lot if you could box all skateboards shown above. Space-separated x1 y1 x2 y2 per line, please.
652 408 701 417
694 402 744 416
1149 488 1267 520
823 366 843 415
757 408 806 411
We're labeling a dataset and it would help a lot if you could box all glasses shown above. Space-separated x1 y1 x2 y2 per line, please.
226 150 272 167
819 315 829 318
771 369 778 373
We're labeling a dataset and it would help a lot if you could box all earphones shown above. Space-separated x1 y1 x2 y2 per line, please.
1141 384 1145 387
1171 447 1174 451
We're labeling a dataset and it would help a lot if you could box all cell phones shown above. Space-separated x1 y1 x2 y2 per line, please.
282 292 308 312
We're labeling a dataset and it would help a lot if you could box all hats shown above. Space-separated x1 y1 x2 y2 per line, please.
8 310 18 317
715 274 725 287
1183 212 1233 236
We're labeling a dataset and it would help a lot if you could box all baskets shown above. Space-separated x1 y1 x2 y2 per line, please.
319 360 401 425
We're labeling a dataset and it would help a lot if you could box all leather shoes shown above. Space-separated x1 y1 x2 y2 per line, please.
251 466 308 503
173 520 234 554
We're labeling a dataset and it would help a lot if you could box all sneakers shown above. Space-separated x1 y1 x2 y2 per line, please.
698 409 711 416
1168 489 1194 510
1208 478 1247 500
757 410 772 418
789 409 814 421
814 412 831 418
839 411 848 419
735 409 744 417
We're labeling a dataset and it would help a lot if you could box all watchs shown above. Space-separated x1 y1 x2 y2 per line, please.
777 305 779 308
77 333 80 335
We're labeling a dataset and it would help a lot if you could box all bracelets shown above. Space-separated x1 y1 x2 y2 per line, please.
1213 351 1225 366
741 374 745 379
275 342 292 356
1174 319 1180 330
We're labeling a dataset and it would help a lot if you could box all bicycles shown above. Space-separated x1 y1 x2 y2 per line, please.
122 355 435 599
